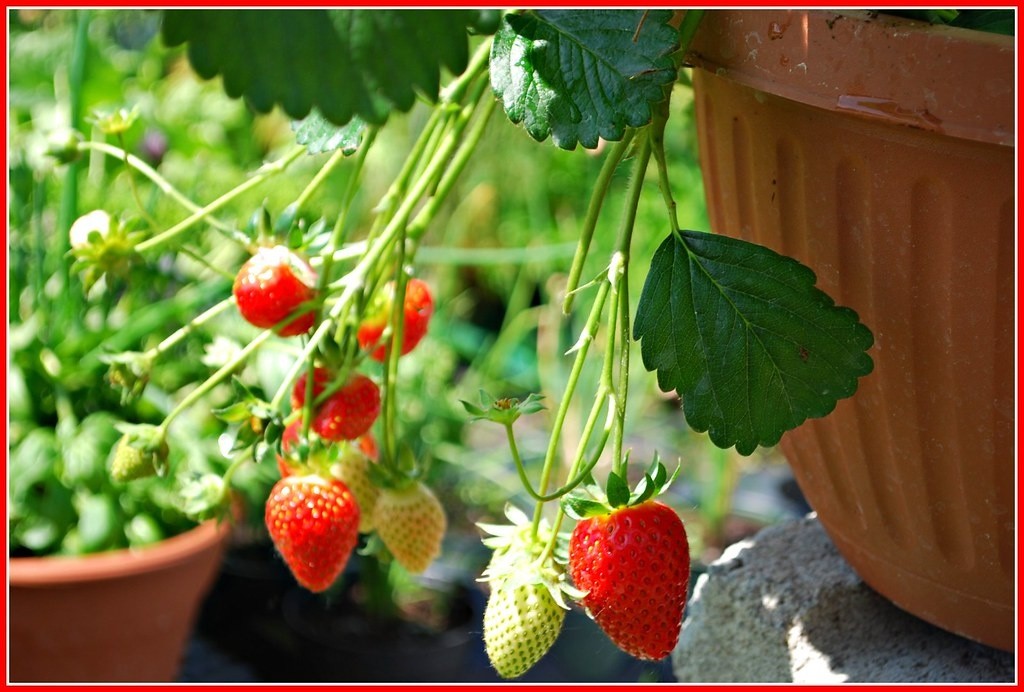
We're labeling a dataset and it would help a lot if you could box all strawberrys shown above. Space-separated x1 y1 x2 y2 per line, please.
65 211 691 680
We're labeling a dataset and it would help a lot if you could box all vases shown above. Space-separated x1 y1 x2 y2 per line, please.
9 482 240 681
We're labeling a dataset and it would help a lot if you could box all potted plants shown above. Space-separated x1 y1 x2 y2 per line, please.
36 12 1017 680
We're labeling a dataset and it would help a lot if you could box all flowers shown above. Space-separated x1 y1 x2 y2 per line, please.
7 54 317 556
